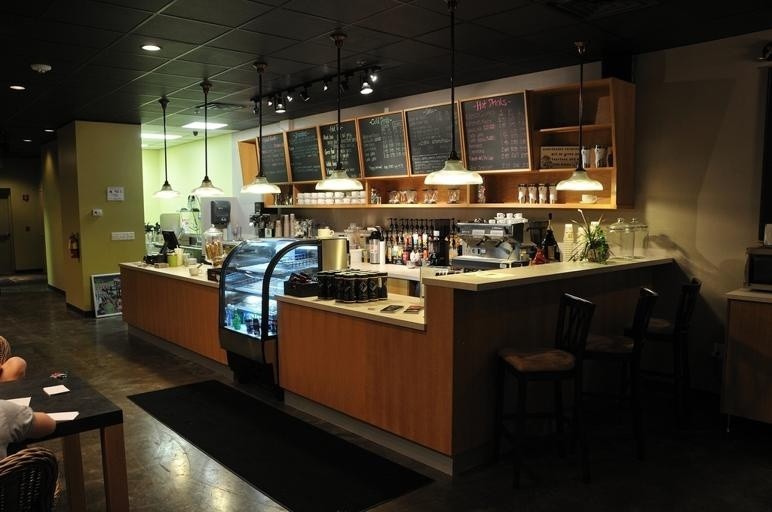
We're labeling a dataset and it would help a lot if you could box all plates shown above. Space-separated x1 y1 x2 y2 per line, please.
579 201 598 203
315 236 338 240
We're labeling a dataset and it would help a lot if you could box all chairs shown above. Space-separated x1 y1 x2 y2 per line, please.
577 285 658 426
497 294 596 440
626 274 702 398
0 446 56 512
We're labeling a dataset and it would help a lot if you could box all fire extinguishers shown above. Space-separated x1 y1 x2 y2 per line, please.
69 232 79 259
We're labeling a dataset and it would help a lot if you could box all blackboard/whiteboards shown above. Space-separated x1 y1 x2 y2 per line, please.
285 124 325 184
404 100 465 178
460 90 532 174
356 110 410 179
318 119 362 184
255 132 290 186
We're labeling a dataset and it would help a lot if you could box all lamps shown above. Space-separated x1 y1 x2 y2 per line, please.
239 62 280 195
423 0 485 185
315 27 365 191
554 38 604 192
191 76 225 196
152 95 179 199
249 65 382 113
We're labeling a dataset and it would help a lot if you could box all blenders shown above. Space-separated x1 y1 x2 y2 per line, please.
527 218 548 258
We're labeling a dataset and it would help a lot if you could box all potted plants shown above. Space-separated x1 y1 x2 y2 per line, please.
561 208 616 266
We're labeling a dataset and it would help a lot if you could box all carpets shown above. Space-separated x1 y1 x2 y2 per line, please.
118 379 451 512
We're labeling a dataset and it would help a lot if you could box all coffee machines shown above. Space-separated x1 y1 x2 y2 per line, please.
453 222 524 270
247 201 278 239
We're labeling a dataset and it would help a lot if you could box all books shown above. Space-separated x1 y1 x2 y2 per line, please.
380 305 404 313
404 305 422 314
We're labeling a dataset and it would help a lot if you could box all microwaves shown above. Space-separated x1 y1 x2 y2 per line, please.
742 244 772 293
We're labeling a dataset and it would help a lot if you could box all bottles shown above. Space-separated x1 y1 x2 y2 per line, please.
369 183 462 205
202 222 225 263
317 266 389 306
626 218 651 259
225 304 276 336
517 183 559 204
385 216 457 266
369 232 380 265
608 217 635 260
763 223 772 248
581 144 612 168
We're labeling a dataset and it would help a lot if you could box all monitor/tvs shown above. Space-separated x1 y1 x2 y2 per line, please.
162 231 180 250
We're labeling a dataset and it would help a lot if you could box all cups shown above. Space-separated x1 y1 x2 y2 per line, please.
167 246 204 276
494 212 523 219
562 221 600 263
582 194 598 202
274 214 295 238
297 191 366 205
318 229 335 237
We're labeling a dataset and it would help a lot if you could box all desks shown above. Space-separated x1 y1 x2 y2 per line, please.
1 372 128 512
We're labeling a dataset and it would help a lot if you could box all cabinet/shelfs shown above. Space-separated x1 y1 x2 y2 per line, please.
237 136 260 186
528 78 636 171
721 286 772 433
262 171 635 209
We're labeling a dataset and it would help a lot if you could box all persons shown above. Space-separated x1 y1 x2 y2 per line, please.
0 335 27 382
1 398 56 460
